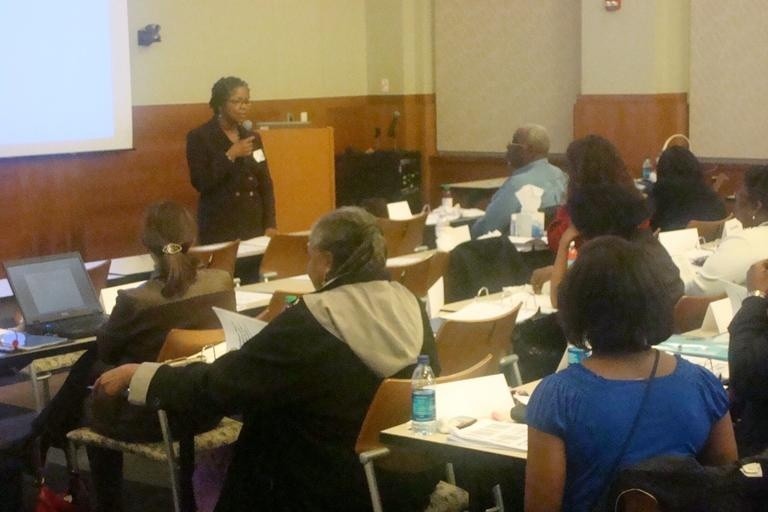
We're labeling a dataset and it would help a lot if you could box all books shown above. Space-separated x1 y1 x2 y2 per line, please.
13 333 69 352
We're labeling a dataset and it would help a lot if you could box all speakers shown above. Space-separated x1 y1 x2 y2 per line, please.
335 150 422 215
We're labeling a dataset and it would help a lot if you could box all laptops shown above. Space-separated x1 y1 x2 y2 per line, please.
2 250 112 339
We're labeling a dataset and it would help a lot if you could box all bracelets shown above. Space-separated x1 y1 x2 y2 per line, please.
747 288 768 301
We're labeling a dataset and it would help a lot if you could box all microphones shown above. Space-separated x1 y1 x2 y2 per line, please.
241 120 254 165
387 110 401 137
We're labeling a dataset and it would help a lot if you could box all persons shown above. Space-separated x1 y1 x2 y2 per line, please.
545 133 654 259
448 123 570 297
89 201 443 511
185 75 278 286
1 198 241 511
681 164 768 301
526 171 686 318
519 237 741 511
723 256 767 459
644 144 728 234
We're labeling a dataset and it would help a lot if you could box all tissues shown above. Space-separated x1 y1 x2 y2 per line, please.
508 183 546 239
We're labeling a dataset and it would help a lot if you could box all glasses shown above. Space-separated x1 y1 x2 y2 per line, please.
228 98 254 107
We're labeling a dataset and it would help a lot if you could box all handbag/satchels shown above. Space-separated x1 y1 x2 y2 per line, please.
31 432 94 512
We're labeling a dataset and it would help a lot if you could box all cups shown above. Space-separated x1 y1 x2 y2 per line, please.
300 112 308 121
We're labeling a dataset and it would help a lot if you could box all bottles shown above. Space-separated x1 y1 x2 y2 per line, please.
567 340 594 370
641 155 651 177
439 183 452 214
411 354 438 433
568 242 577 265
